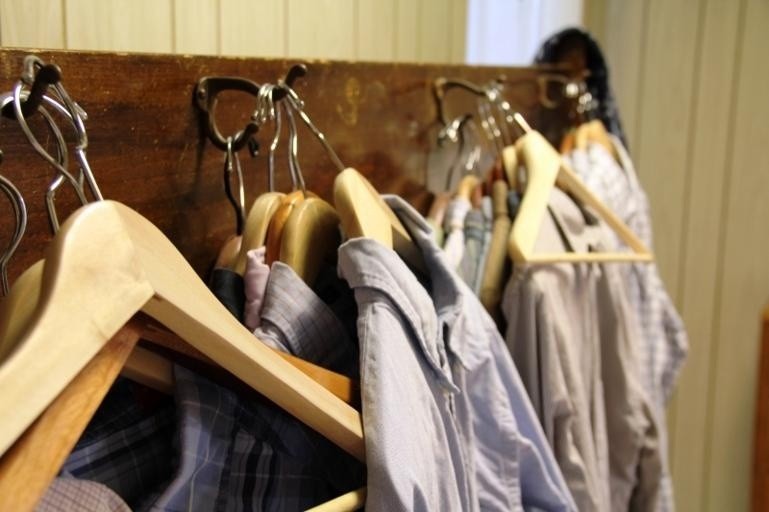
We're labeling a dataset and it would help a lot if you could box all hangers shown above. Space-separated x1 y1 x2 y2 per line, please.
0 54 486 512
426 76 652 322
